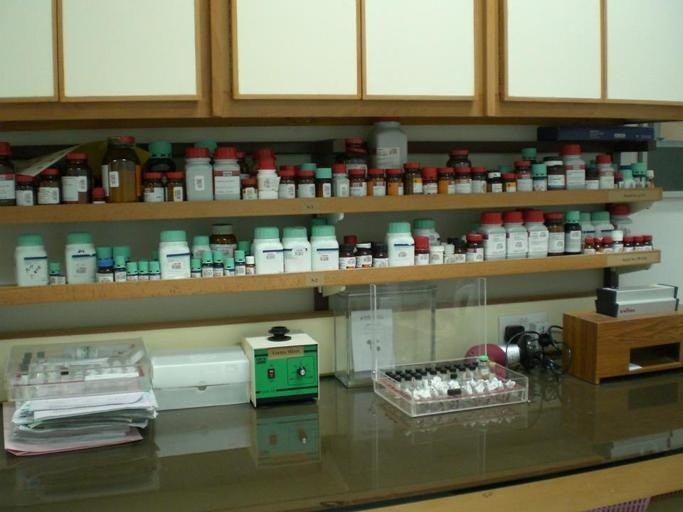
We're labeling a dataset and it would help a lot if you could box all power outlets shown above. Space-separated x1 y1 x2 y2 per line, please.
524 312 551 349
497 314 527 348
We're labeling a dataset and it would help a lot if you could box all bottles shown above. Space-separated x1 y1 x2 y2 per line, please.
384 355 495 400
13 205 651 289
0 117 646 205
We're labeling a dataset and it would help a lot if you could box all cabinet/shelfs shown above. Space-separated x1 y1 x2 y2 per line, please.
0 186 661 305
0 1 219 121
209 1 492 121
486 0 681 123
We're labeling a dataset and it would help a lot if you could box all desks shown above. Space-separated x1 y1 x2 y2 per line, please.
0 355 683 508
